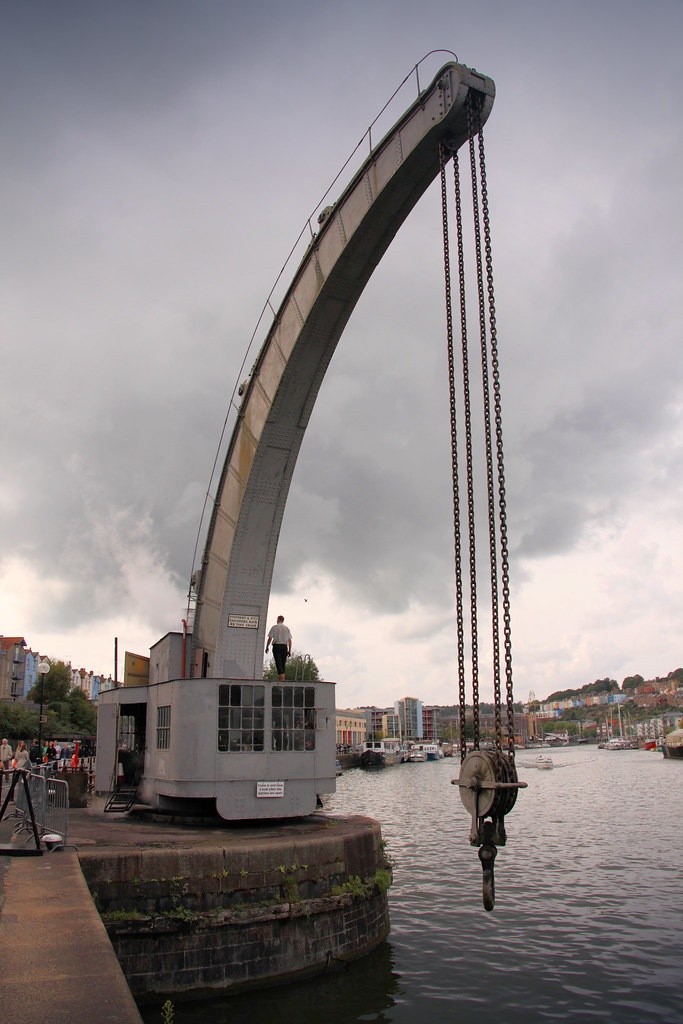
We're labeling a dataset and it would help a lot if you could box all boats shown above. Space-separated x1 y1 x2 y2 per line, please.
334 759 344 777
355 738 555 771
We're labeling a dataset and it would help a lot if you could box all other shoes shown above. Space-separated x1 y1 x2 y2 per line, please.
277 676 284 682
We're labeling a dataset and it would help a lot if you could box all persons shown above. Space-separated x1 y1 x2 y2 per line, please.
336 743 351 754
0 738 12 783
43 741 48 752
14 740 29 769
53 740 61 760
29 741 44 765
264 615 292 681
60 743 70 765
29 737 38 750
296 709 314 752
46 741 57 762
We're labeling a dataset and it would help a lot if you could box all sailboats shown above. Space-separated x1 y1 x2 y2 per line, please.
597 699 683 760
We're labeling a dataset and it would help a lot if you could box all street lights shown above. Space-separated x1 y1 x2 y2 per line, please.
36 662 50 763
